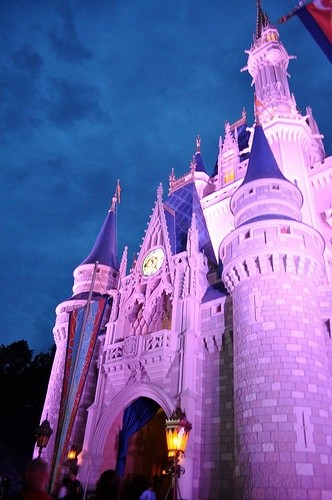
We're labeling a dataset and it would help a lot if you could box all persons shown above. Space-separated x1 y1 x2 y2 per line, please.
139 484 156 500
53 465 85 500
17 458 55 500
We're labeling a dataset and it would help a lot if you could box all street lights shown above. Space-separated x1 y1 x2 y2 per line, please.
160 395 193 500
34 411 54 458
67 442 77 472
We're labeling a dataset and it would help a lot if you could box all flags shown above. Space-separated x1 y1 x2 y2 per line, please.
295 0 332 64
